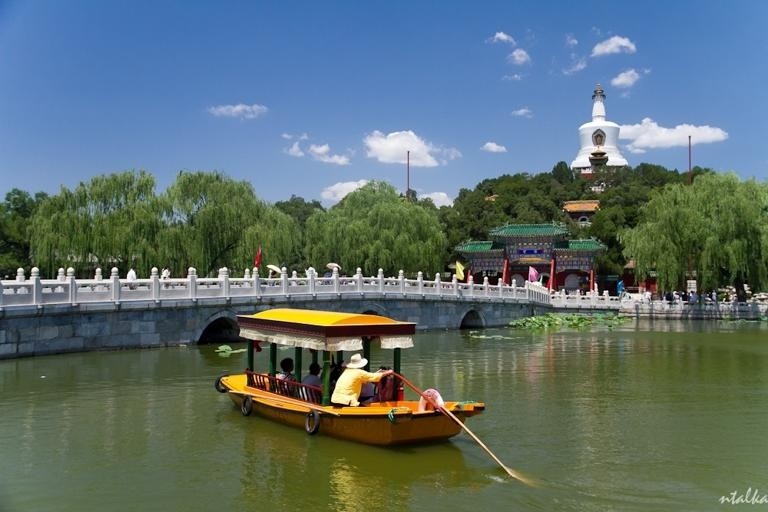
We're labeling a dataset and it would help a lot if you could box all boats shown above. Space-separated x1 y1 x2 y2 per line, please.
219 308 485 447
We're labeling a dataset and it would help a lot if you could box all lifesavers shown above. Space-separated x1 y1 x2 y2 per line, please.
419 389 444 412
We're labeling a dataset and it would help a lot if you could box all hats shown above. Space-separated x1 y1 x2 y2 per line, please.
344 353 369 369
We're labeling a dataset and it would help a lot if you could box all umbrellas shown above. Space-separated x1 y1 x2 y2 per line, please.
265 264 283 274
325 262 344 270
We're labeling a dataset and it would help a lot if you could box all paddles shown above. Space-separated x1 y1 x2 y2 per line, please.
381 369 533 486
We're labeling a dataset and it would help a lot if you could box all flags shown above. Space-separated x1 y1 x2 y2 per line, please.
455 261 466 281
528 266 540 283
254 245 263 267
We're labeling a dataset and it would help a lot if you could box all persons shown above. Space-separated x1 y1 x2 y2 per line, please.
273 357 297 397
329 359 405 407
657 288 719 306
329 352 395 408
322 268 333 286
299 361 323 403
269 270 278 287
